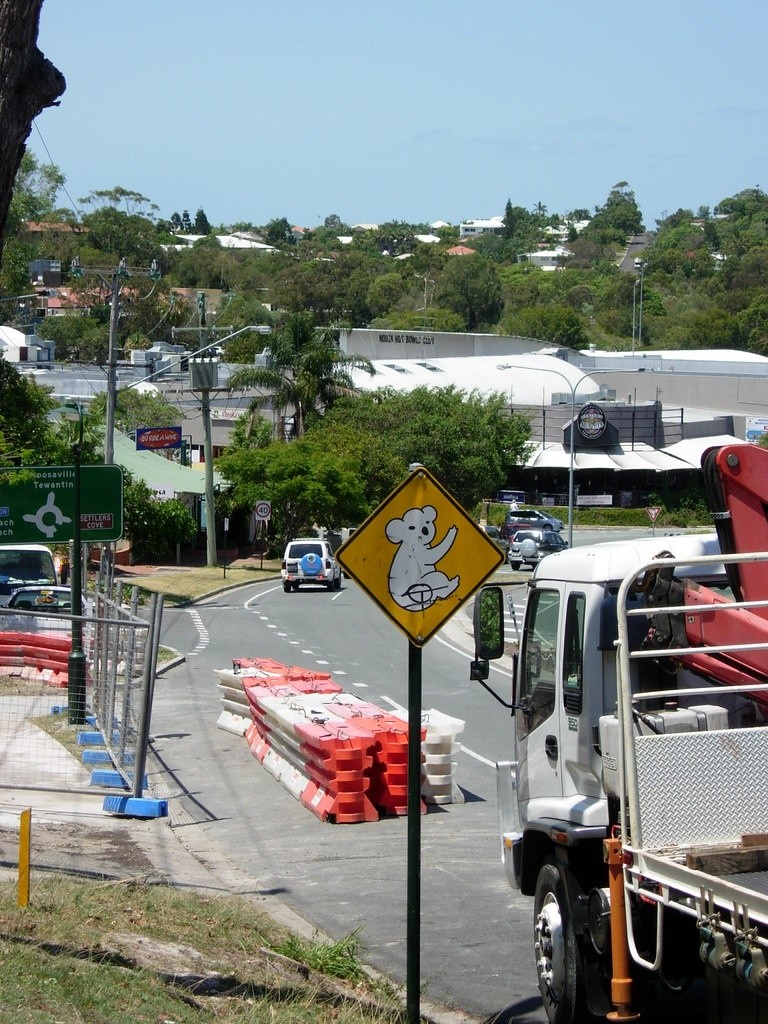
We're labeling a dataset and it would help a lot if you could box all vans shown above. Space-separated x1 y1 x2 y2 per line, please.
505 510 565 533
1 545 58 590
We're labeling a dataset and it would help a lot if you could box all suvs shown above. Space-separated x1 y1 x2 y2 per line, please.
508 530 569 571
281 539 341 592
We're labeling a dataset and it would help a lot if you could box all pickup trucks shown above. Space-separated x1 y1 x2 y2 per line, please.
0 587 97 646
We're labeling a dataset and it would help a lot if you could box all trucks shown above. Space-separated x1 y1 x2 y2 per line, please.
470 530 768 1024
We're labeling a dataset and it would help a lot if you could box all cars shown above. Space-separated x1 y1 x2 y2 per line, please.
500 523 533 544
479 525 510 566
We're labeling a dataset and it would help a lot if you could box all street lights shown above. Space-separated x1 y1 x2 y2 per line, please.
104 324 272 467
497 363 655 551
